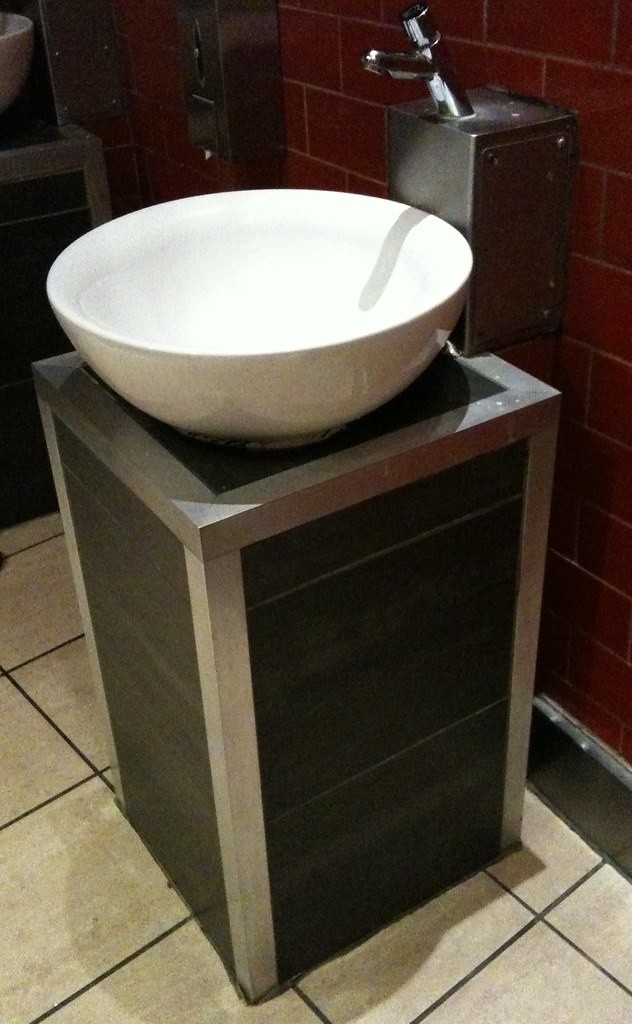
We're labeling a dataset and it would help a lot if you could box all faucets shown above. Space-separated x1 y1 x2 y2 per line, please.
359 3 476 119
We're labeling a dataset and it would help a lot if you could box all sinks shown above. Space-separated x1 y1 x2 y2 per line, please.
41 185 475 443
0 11 35 115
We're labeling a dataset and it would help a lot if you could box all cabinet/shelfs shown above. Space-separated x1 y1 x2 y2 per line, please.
23 352 562 1004
0 109 105 528
